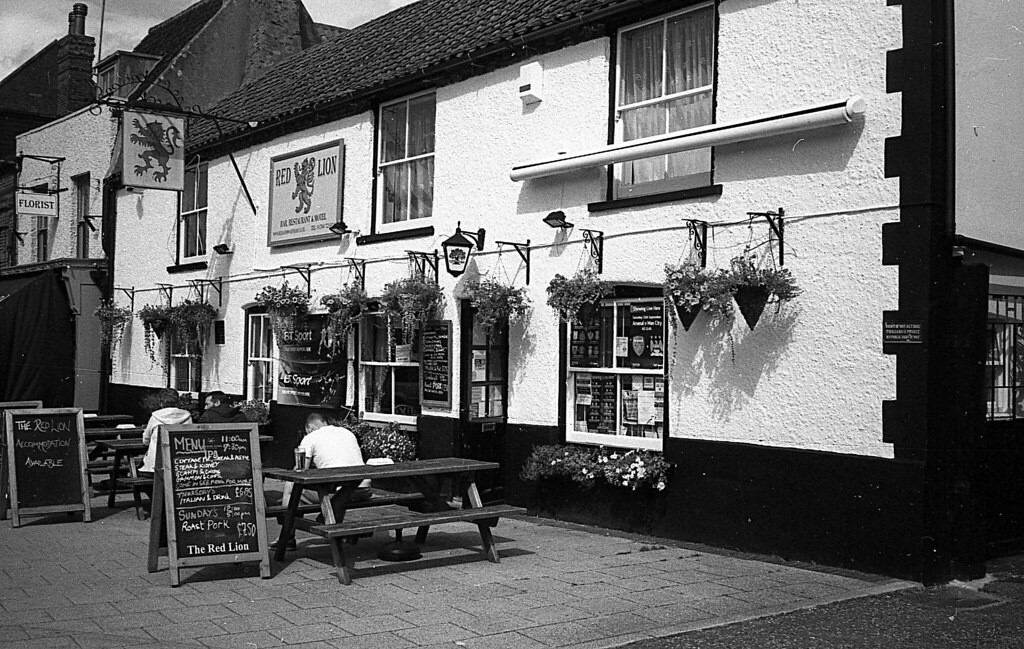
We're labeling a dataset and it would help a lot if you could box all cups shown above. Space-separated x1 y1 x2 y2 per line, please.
294 447 306 472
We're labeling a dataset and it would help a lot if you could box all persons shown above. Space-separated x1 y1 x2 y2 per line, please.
268 413 372 552
138 389 248 502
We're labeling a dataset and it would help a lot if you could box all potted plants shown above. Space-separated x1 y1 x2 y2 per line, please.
136 300 218 378
94 297 133 374
549 268 616 339
462 276 533 344
381 269 447 354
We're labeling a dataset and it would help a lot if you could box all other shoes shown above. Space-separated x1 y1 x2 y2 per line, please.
268 537 297 551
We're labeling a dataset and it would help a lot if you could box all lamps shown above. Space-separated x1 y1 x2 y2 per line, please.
330 222 359 235
440 221 485 277
212 243 234 254
543 211 575 232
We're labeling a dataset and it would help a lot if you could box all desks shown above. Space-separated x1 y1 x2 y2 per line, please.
263 458 500 585
82 415 134 461
97 435 274 522
84 426 144 487
95 438 149 522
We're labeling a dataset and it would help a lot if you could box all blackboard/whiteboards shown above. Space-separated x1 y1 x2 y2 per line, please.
147 424 273 567
0 401 90 512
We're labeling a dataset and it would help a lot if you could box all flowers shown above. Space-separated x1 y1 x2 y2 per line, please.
318 275 369 361
664 256 805 384
257 281 317 358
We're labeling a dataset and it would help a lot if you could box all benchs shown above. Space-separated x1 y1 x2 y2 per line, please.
310 503 527 580
85 441 153 521
262 491 449 563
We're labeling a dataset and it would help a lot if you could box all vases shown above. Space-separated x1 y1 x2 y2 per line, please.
732 291 771 332
673 295 702 331
